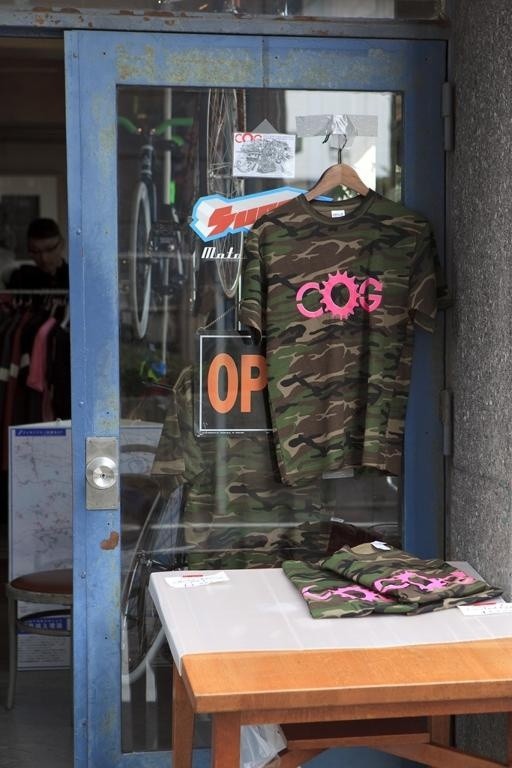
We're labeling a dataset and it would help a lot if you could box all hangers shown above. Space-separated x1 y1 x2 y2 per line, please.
1 289 69 330
300 133 369 204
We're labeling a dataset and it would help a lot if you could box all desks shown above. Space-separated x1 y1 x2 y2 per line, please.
146 559 512 768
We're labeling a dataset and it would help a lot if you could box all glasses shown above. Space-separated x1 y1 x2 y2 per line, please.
27 243 60 254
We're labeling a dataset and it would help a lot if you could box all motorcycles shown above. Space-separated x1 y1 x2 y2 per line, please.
329 514 401 548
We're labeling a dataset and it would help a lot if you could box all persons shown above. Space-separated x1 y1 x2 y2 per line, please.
8 218 69 290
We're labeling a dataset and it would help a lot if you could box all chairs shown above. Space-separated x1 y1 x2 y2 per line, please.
2 443 172 712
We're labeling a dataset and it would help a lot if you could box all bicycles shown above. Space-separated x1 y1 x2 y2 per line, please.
118 107 204 338
205 86 253 300
121 482 185 683
